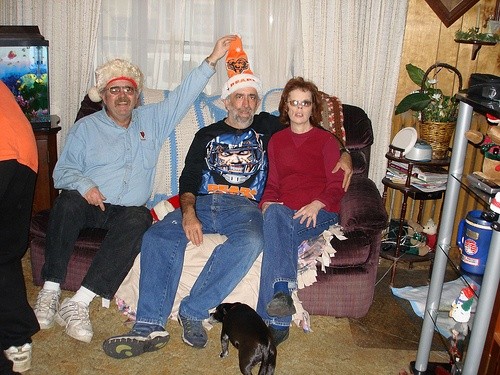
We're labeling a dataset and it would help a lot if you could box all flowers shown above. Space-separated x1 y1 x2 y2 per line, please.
394 63 459 123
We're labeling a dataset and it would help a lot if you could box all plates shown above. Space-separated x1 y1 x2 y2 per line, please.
391 126 418 157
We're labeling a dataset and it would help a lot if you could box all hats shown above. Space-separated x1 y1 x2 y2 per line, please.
87 58 143 102
221 32 261 99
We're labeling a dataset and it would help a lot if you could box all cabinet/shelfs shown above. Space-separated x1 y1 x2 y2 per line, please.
380 72 500 375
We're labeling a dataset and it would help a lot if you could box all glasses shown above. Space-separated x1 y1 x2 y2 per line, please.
102 86 136 95
287 100 314 106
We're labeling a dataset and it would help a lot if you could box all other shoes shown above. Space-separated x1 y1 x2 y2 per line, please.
268 325 289 347
266 293 295 317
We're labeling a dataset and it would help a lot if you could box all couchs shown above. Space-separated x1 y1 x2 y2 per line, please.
29 86 389 317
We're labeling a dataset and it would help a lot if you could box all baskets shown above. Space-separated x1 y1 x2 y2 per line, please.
418 61 462 159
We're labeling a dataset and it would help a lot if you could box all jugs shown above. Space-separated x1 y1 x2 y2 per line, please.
456 210 495 274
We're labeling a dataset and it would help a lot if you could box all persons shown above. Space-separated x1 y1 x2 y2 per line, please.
103 35 368 359
258 76 347 348
32 35 234 343
0 76 38 375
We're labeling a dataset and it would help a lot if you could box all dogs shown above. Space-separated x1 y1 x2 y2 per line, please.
208 300 277 375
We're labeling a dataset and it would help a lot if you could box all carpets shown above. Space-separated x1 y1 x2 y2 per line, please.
0 244 459 375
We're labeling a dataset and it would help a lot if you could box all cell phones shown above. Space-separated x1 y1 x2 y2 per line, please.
467 173 500 194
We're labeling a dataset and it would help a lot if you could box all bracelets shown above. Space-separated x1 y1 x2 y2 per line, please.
206 59 217 66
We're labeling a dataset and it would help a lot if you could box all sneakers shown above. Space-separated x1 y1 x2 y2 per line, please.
55 297 93 343
4 342 31 372
177 312 207 348
103 323 169 358
32 288 62 328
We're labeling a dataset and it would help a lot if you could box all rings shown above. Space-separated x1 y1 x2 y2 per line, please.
309 215 312 218
